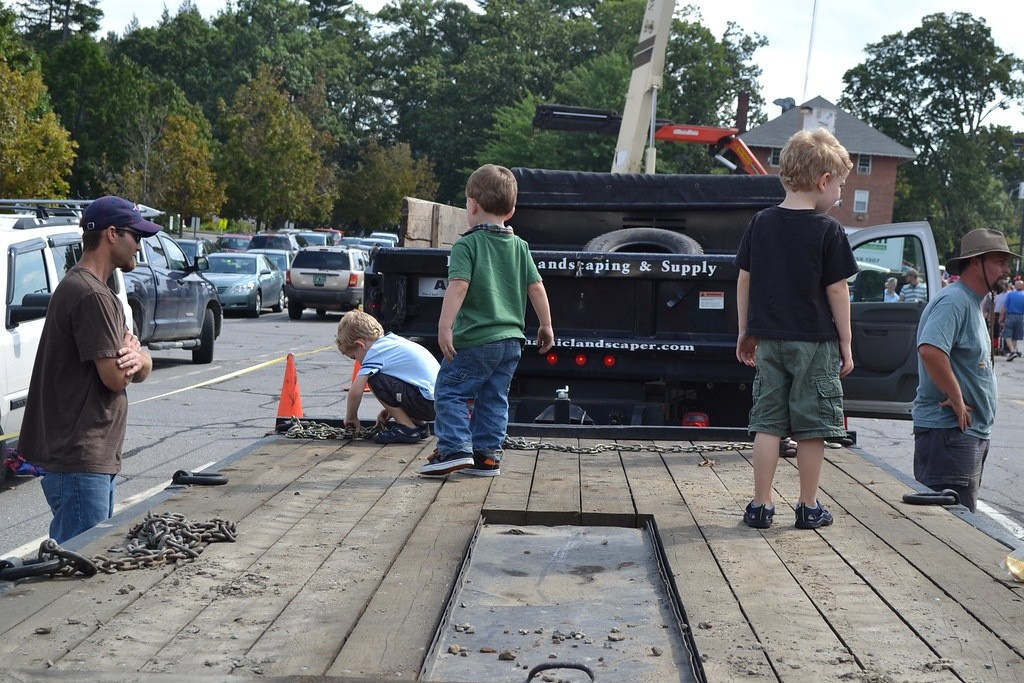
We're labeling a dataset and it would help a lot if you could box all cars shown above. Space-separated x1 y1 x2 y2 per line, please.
175 228 400 320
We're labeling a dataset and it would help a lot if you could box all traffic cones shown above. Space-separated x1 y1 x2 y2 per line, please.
343 359 371 393
266 352 305 435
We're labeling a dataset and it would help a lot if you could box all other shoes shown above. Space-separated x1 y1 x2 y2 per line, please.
1007 352 1018 361
1016 351 1022 357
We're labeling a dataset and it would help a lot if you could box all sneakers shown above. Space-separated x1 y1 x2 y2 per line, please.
420 448 474 475
795 498 833 529
452 449 505 477
744 499 776 528
415 422 430 439
373 423 421 444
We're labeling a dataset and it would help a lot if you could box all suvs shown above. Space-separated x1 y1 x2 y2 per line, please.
0 198 167 441
123 230 224 364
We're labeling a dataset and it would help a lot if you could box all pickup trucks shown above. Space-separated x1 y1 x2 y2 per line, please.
359 166 945 430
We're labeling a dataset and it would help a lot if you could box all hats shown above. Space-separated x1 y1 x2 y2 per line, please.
79 196 163 237
902 268 918 277
950 275 960 283
946 228 1024 275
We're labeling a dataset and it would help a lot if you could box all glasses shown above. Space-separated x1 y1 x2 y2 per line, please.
106 226 143 243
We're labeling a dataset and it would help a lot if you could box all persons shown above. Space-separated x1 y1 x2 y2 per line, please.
419 164 555 477
732 125 859 531
882 264 1024 362
17 196 163 545
910 227 1023 515
335 308 441 444
